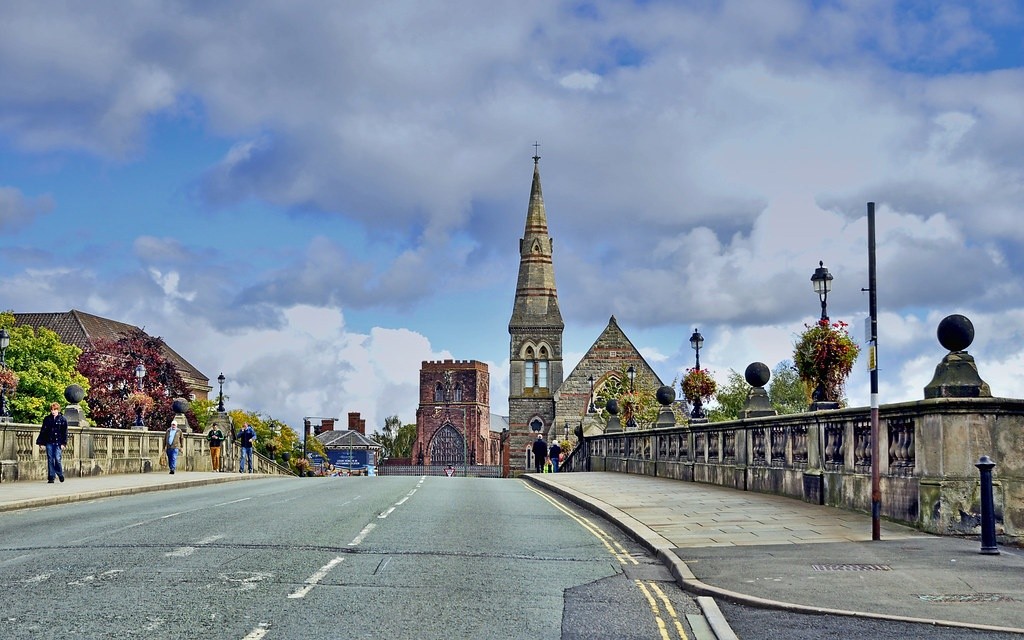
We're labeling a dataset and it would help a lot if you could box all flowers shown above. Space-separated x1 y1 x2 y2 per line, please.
681 367 716 403
791 318 857 383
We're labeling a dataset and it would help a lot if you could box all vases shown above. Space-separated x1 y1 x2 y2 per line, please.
690 398 703 418
811 381 827 401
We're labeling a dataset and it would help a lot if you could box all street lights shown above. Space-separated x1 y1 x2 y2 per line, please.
625 364 638 427
810 261 839 410
587 374 597 413
269 424 276 460
563 423 570 460
0 326 10 417
136 365 146 426
217 372 226 411
689 328 708 424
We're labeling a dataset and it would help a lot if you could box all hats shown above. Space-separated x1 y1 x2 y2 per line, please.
171 421 177 425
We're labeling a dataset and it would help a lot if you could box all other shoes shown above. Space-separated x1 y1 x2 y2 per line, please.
248 469 253 473
48 479 54 483
239 469 242 473
169 470 174 474
59 476 64 482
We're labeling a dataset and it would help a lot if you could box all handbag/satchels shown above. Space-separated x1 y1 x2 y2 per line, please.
160 450 167 467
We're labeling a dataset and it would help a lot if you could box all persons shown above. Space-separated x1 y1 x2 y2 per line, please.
162 421 183 474
36 402 67 483
206 422 224 473
533 434 561 474
237 422 256 474
